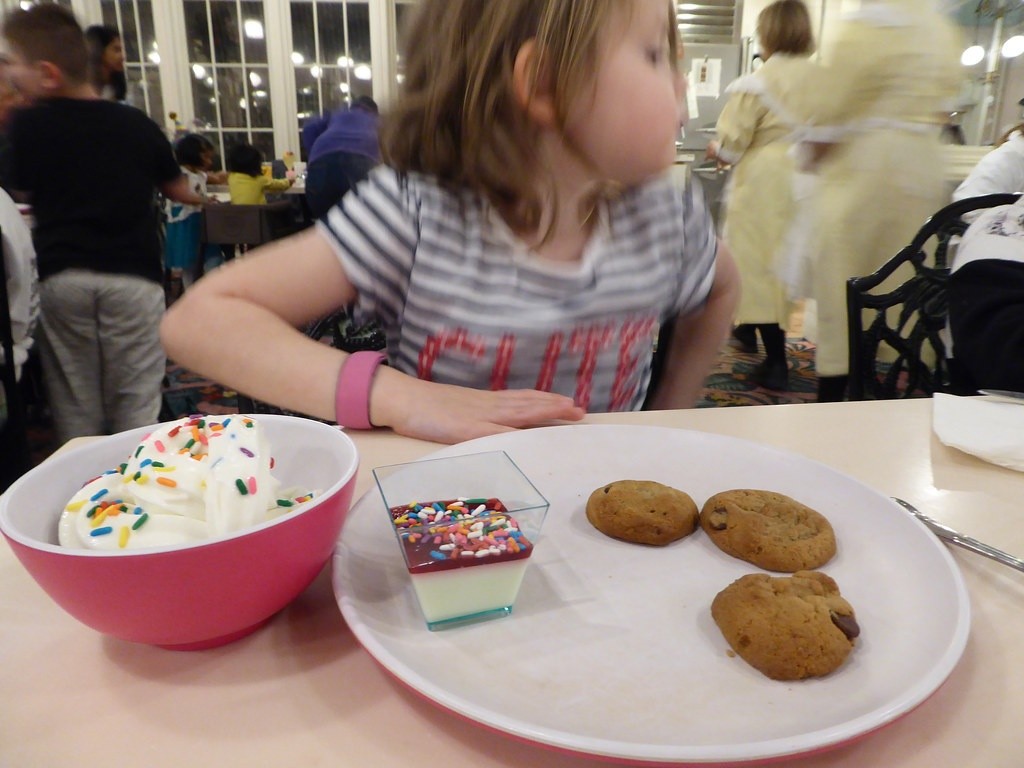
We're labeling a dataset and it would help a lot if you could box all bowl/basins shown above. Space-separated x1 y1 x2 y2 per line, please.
0 414 360 650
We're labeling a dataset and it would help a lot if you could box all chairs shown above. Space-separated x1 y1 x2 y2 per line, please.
846 193 1020 402
206 203 276 268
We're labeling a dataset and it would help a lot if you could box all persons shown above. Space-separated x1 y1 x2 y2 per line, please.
0 0 384 438
704 0 1024 402
159 0 743 445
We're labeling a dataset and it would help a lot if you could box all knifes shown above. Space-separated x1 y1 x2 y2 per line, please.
890 495 1023 572
977 389 1024 400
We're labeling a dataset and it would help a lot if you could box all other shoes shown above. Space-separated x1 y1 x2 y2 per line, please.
732 327 758 347
751 360 789 392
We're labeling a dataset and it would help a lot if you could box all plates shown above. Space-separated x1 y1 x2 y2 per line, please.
332 423 970 768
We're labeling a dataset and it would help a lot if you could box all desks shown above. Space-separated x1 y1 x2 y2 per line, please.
264 185 311 240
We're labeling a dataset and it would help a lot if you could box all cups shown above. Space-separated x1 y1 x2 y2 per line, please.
371 449 551 633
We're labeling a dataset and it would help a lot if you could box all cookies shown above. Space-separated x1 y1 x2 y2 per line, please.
710 569 860 680
585 479 699 545
700 488 837 573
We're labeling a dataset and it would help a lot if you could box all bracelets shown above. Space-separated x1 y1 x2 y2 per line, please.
334 349 393 431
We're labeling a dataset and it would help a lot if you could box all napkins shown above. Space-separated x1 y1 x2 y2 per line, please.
934 390 1022 475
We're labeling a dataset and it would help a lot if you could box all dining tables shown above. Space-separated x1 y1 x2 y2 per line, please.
0 404 1023 767
